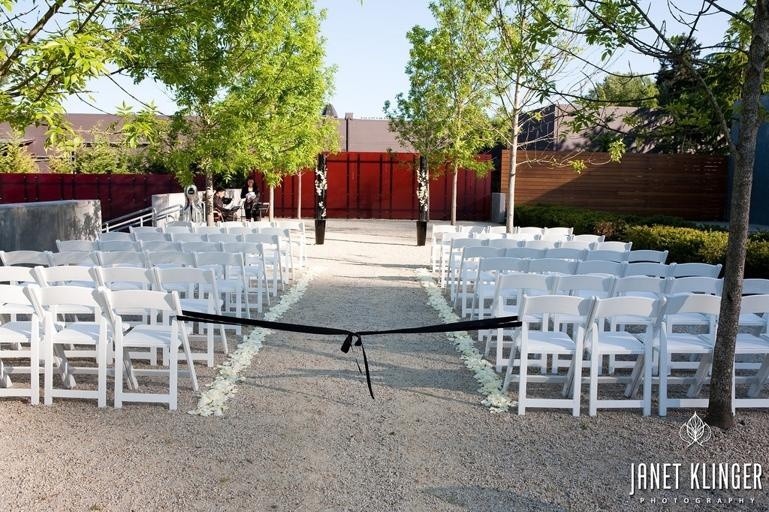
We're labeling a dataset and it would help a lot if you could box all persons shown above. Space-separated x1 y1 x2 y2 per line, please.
202 176 263 222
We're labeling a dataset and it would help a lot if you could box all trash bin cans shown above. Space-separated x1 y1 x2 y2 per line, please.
492 193 506 222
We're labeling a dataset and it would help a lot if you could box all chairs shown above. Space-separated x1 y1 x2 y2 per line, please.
430 221 769 419
0 215 308 413
